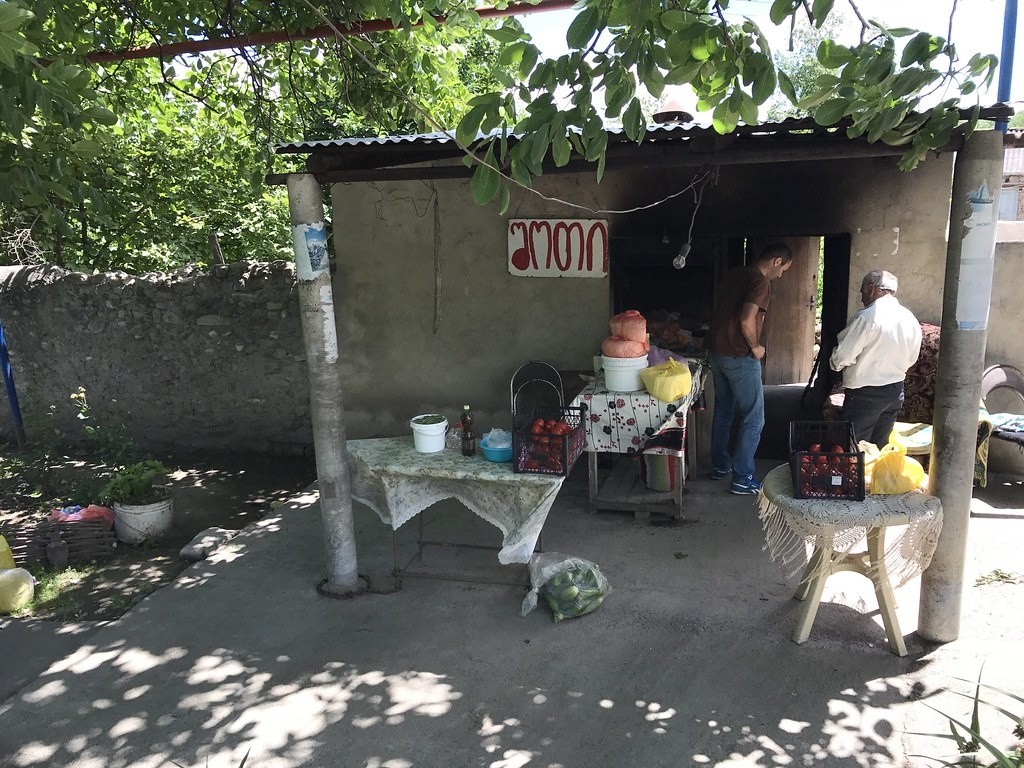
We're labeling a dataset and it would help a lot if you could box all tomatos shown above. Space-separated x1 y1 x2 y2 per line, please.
796 443 858 495
526 418 583 470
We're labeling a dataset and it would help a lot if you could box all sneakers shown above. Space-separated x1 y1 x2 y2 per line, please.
729 475 763 495
712 464 733 480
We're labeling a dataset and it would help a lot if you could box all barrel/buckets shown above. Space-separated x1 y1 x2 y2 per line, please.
601 353 648 391
113 486 174 544
410 414 449 453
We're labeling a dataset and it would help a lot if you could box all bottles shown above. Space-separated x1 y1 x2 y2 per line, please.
460 405 475 457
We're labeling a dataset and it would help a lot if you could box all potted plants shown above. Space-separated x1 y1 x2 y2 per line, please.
96 460 175 545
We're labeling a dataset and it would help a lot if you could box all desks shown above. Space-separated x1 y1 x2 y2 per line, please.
562 358 710 523
346 434 583 591
753 462 943 658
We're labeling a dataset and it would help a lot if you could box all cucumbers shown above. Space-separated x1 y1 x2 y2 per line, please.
541 564 607 622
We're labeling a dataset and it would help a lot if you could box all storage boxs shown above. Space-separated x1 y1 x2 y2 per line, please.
513 403 588 477
788 420 865 502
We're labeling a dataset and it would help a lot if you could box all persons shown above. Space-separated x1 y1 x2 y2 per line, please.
705 241 793 497
828 268 922 452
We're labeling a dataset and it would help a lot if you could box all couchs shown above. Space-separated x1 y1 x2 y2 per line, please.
824 321 993 488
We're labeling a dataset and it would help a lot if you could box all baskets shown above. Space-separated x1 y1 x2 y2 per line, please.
789 421 865 501
512 402 588 478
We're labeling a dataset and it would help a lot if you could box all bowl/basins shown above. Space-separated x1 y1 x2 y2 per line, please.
480 434 513 462
